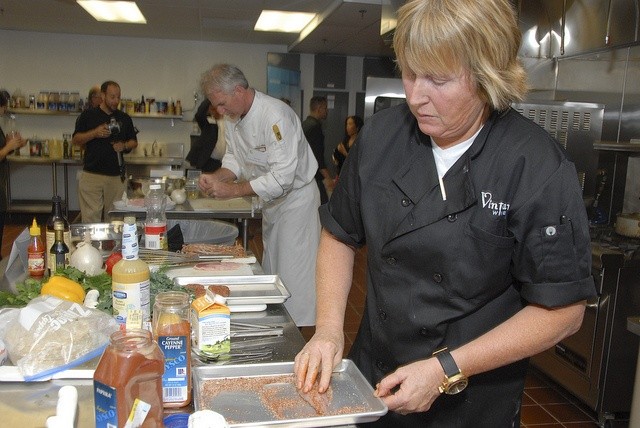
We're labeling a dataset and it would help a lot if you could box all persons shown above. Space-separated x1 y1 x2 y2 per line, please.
332 116 364 176
72 81 138 224
199 64 321 331
280 98 290 107
301 96 336 204
0 90 28 259
86 85 102 108
195 99 226 169
294 0 597 428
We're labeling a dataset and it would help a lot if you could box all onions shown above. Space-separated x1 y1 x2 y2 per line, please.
70 245 107 277
170 188 187 205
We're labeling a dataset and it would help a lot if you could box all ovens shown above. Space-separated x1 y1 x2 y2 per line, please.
530 248 640 428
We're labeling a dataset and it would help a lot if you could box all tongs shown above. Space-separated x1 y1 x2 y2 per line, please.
230 322 284 338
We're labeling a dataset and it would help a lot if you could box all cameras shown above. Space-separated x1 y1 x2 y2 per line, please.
108 117 122 137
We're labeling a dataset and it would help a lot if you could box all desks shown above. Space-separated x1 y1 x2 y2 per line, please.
6 143 184 218
107 176 263 252
0 250 358 428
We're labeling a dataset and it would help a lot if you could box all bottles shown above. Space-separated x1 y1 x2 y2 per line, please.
78 99 84 111
154 291 191 407
111 217 151 333
175 100 182 115
46 195 71 275
64 139 68 158
62 134 65 158
50 223 69 276
145 185 169 250
29 95 35 109
44 139 50 158
20 97 24 107
121 99 126 112
145 101 149 113
140 94 145 112
135 100 140 112
92 327 164 428
150 99 158 113
28 218 45 274
68 134 72 157
16 97 21 107
85 97 88 107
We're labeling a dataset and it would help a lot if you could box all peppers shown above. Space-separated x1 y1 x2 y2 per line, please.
41 275 86 305
105 253 121 276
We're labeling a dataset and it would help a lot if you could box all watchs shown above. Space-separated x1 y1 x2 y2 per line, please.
432 347 468 395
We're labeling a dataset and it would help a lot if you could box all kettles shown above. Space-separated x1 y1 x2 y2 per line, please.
11 95 15 108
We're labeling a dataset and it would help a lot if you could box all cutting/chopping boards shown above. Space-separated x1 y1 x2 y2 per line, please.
189 196 252 213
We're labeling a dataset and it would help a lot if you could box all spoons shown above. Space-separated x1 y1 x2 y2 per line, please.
195 357 271 365
191 347 271 359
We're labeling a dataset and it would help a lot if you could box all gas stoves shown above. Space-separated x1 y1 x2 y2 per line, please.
585 223 640 249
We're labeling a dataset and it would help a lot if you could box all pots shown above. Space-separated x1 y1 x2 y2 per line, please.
119 219 240 245
613 212 640 239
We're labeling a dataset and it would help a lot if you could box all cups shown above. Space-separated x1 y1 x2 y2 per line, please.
186 170 202 199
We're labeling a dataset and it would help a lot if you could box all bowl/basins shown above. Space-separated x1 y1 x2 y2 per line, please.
67 219 141 251
